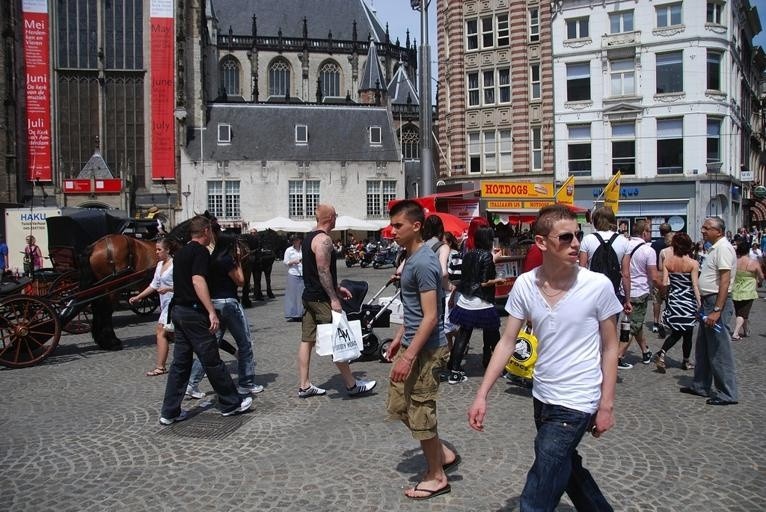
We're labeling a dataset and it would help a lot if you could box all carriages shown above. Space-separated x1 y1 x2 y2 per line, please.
0 206 222 371
44 214 288 317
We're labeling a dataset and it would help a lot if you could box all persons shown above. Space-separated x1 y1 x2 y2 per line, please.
397 214 508 384
523 205 764 405
300 205 377 397
0 234 8 280
284 235 307 322
24 235 43 272
129 215 264 427
330 234 400 256
381 199 462 503
466 202 626 511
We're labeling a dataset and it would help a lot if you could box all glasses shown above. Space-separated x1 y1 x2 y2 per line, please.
540 230 585 244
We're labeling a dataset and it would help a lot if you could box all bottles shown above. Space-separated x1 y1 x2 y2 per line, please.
500 243 529 257
698 312 724 334
620 312 631 343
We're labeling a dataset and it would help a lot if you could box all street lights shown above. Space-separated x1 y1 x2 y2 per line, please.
411 1 433 195
705 161 725 220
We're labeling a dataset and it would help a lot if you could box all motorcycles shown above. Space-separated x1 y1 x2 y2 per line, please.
343 239 406 271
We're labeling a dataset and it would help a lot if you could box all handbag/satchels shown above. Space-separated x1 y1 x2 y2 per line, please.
504 322 539 387
378 296 405 326
315 308 365 356
448 286 458 310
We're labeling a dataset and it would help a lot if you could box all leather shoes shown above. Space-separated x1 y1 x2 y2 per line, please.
680 384 738 405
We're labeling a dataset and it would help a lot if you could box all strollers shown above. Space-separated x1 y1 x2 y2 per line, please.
333 273 401 363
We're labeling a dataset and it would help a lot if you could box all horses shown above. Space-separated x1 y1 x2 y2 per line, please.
78 210 222 351
221 228 293 309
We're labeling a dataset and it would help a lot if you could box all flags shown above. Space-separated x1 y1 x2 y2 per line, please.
553 175 575 207
603 170 621 215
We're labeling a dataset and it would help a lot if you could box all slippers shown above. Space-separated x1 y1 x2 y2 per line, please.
405 478 450 500
731 334 743 341
439 454 462 473
743 329 752 337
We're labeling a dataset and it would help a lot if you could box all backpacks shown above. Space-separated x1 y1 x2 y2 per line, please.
24 256 32 274
590 230 621 293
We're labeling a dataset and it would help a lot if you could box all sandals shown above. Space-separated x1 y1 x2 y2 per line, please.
145 367 167 376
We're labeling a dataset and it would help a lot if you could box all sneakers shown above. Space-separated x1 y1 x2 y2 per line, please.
439 372 448 383
652 322 660 334
219 396 253 418
656 350 667 373
237 383 263 396
184 383 207 399
347 380 377 395
615 360 633 371
448 370 468 384
642 348 653 365
159 411 189 425
298 384 326 398
